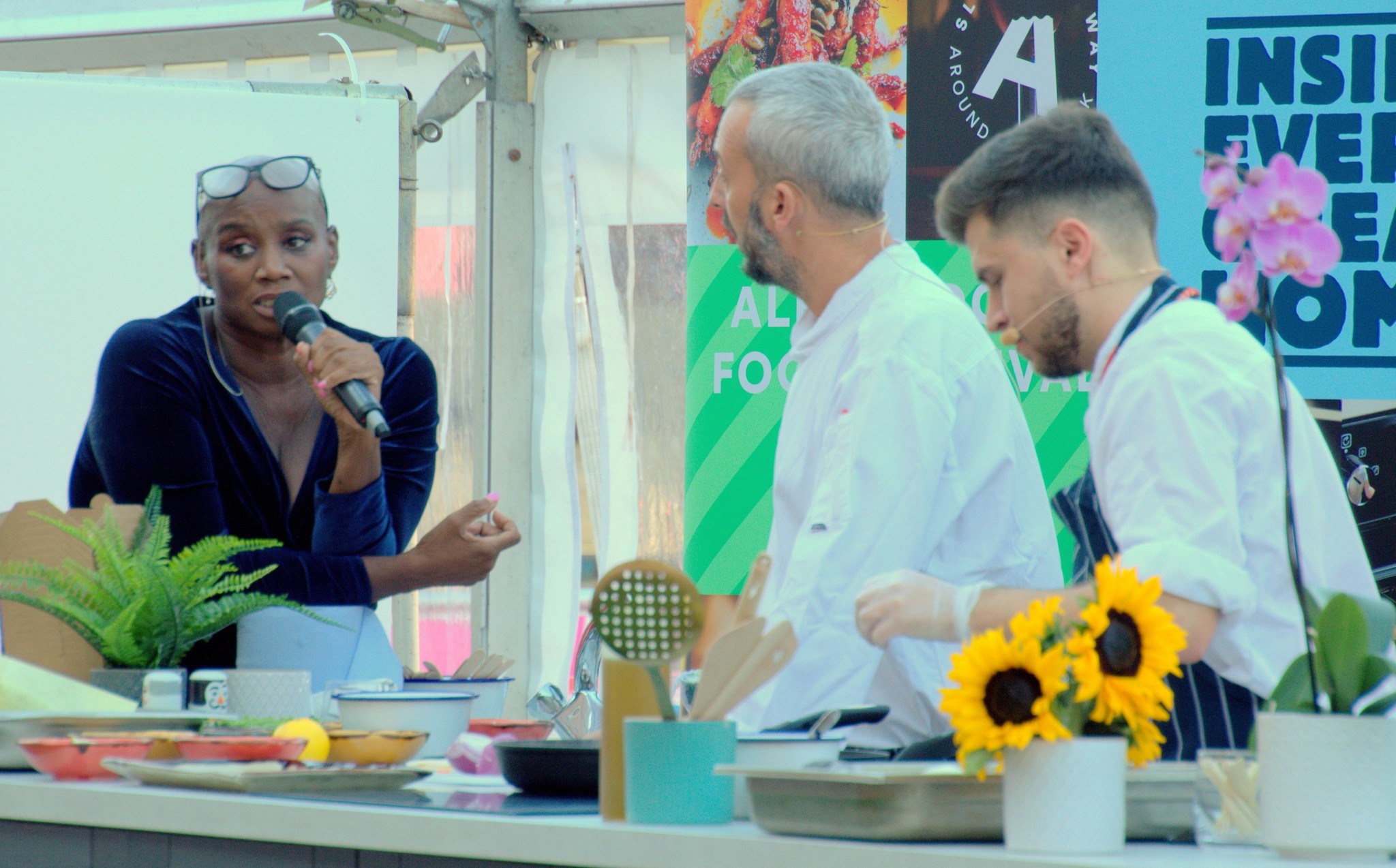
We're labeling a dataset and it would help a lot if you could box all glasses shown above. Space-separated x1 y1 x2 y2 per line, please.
195 155 329 234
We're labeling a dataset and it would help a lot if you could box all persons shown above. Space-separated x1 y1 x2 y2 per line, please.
856 98 1396 762
708 60 1066 760
68 154 521 709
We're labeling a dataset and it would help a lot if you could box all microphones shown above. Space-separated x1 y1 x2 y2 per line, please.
272 291 392 440
998 268 1147 345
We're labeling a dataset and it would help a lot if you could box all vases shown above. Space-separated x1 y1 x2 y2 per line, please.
1003 736 1128 859
1250 709 1396 863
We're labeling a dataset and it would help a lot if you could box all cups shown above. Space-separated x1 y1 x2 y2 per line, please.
1190 749 1262 853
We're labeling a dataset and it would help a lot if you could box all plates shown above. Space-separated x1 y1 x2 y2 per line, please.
99 757 432 795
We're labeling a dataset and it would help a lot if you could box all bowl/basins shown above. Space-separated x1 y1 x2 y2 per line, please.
15 738 151 782
332 690 479 757
403 676 516 717
493 739 601 801
468 718 556 740
328 731 430 769
173 737 307 760
732 732 846 820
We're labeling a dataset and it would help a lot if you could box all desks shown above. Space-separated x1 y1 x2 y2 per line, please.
0 770 1396 868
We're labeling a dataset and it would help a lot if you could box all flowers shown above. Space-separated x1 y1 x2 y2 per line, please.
1193 141 1396 753
936 552 1189 784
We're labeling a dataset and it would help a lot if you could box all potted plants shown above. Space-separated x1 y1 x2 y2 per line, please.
0 485 356 708
